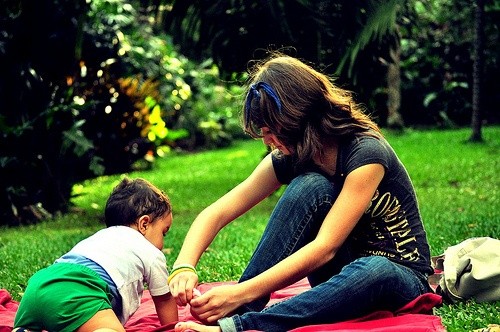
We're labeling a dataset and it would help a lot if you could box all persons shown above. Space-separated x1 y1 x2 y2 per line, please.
12 176 180 331
166 52 441 331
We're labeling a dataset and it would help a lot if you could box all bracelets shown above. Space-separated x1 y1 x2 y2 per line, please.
166 267 195 286
169 263 199 275
